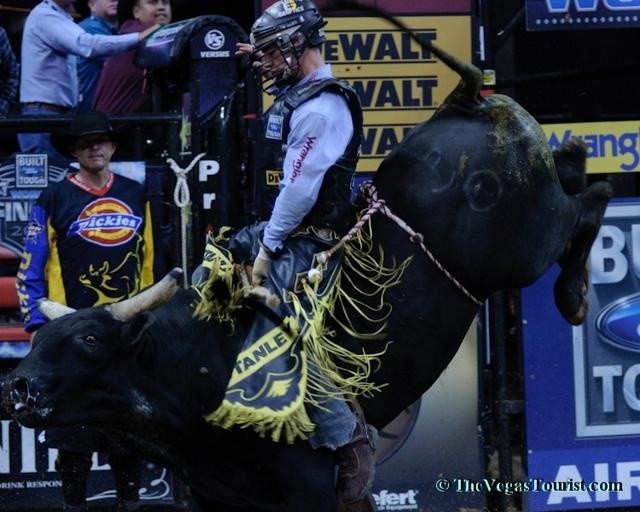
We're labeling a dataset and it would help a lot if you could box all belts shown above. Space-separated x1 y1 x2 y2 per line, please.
23 101 67 114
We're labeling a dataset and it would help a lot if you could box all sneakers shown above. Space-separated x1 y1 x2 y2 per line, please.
338 420 378 506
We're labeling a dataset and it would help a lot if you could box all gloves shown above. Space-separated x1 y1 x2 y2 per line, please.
252 246 274 286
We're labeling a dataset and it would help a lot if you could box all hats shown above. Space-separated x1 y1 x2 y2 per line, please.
49 112 138 159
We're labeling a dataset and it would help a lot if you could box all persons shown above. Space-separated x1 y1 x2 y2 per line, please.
2 0 379 507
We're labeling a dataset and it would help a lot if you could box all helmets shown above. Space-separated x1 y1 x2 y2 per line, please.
249 1 328 95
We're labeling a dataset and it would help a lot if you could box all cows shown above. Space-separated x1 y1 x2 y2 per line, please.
0 0 616 512
